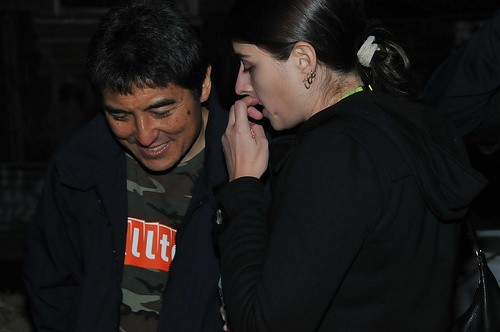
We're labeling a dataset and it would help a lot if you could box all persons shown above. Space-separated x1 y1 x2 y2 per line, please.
14 1 276 332
214 0 500 332
